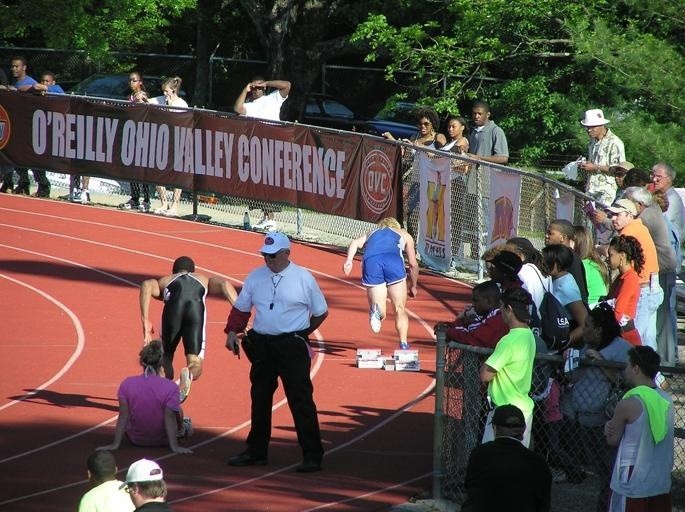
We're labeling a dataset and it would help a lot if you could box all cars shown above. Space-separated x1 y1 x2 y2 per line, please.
368 102 427 139
295 96 357 129
66 70 188 105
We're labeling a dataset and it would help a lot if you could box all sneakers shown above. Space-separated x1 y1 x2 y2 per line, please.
229 454 266 466
176 416 188 437
1 181 183 219
400 342 409 349
253 219 277 233
551 471 567 482
369 303 381 332
298 462 322 472
177 367 191 403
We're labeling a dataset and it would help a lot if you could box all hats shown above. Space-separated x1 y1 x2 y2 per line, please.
609 161 635 177
501 296 532 322
257 231 290 254
604 199 637 217
486 251 523 275
581 109 610 127
118 458 163 490
488 404 524 428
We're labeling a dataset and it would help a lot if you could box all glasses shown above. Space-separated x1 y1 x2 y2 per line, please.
417 122 429 126
611 203 632 213
128 79 141 82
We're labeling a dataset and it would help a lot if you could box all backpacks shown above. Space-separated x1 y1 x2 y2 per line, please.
529 263 571 352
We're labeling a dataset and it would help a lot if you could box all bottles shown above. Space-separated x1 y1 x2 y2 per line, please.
242 212 250 230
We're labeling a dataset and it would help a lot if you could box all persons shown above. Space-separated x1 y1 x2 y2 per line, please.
126 459 170 511
139 256 251 406
433 220 685 510
233 78 292 235
0 68 14 194
224 231 329 473
116 71 153 213
77 450 139 512
0 56 50 198
381 101 509 260
95 339 202 455
136 76 189 217
16 73 92 205
576 108 627 244
592 160 685 376
343 217 419 351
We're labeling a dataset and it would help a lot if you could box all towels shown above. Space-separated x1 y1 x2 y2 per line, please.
623 385 671 445
